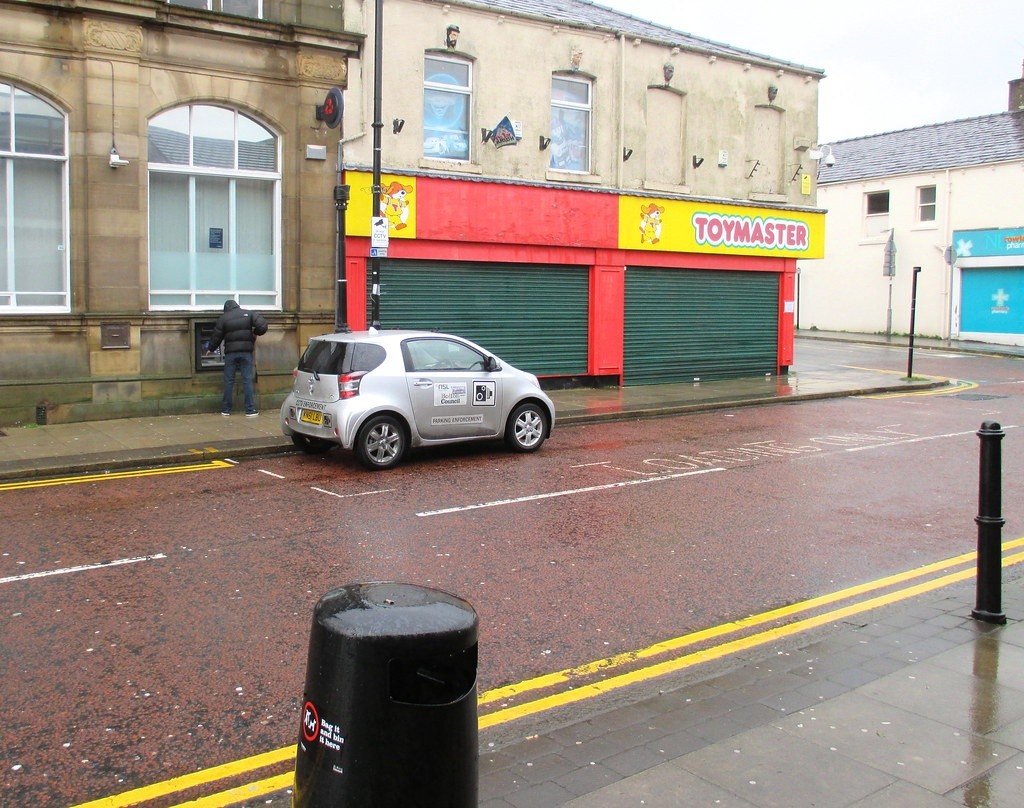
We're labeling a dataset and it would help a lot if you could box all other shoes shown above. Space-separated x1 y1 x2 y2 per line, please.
245 411 259 416
221 412 229 416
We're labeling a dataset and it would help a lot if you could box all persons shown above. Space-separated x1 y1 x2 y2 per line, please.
205 300 268 417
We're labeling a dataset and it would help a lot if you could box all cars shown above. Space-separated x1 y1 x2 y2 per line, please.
280 327 557 471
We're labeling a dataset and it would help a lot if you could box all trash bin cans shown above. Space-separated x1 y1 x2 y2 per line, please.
292 581 479 808
